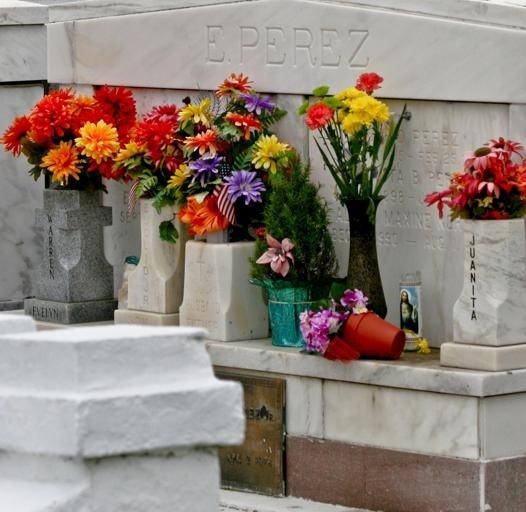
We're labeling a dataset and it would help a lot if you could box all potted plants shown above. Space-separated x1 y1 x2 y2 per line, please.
251 147 338 348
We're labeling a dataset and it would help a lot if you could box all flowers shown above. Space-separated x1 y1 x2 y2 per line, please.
2 82 137 187
296 71 412 226
128 71 288 243
423 136 526 224
298 287 370 354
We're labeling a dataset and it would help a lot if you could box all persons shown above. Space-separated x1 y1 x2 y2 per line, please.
400 289 418 333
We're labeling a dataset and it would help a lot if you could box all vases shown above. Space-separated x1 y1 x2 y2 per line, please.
439 219 525 370
338 194 387 319
24 188 271 342
326 313 404 360
